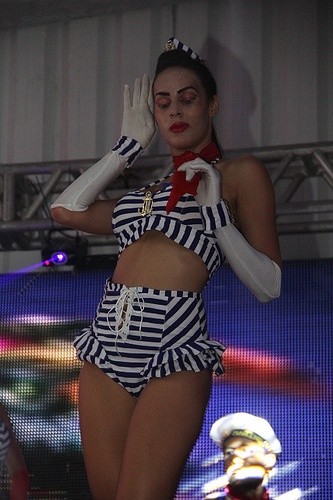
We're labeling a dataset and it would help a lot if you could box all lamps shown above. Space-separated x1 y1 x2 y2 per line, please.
38 236 90 270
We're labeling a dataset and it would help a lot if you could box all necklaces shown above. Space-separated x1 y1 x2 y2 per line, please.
138 165 173 218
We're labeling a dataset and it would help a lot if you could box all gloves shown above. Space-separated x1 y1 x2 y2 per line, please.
177 157 281 301
50 74 155 211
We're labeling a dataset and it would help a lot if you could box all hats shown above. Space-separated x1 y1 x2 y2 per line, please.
209 413 279 449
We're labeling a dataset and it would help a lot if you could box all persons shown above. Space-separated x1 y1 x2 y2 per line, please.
208 413 283 500
49 35 282 500
0 403 29 497
